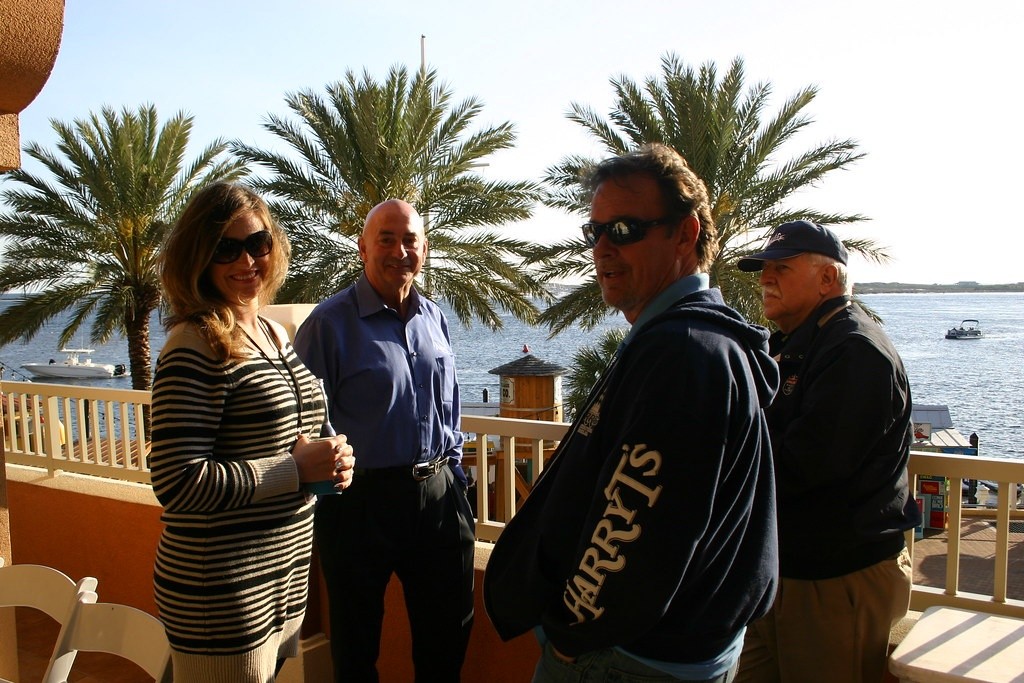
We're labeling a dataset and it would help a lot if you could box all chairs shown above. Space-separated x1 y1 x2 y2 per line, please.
41 591 173 683
0 557 98 683
888 606 1024 683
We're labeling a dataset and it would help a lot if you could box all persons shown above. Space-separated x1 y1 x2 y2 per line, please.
147 181 357 682
483 143 781 683
292 197 478 683
732 221 924 683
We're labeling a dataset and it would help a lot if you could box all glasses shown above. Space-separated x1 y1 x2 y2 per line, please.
211 231 273 264
581 213 681 248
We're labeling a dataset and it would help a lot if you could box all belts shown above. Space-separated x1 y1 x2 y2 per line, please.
350 453 452 482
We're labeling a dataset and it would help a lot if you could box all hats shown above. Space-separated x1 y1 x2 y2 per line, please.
738 221 848 272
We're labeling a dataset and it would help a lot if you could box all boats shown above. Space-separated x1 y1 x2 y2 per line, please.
945 319 982 340
19 349 127 379
